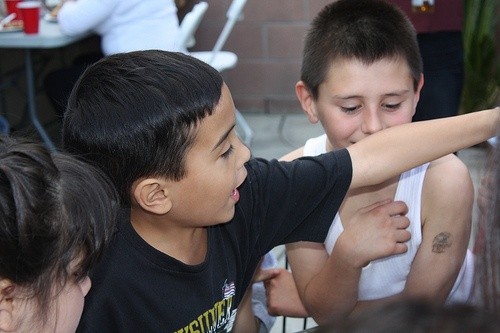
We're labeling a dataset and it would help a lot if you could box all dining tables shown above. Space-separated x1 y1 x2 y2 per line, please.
0 7 95 150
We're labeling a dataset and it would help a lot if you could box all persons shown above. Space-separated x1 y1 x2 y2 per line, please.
56 0 191 57
60 51 500 333
274 0 474 333
0 133 117 333
232 242 321 333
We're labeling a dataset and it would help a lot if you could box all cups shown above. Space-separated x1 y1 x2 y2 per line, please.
15 1 40 34
4 0 24 21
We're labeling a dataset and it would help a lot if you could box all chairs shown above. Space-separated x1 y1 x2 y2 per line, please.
177 0 253 144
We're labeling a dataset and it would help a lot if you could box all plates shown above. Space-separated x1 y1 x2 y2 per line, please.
0 20 24 33
43 13 57 22
